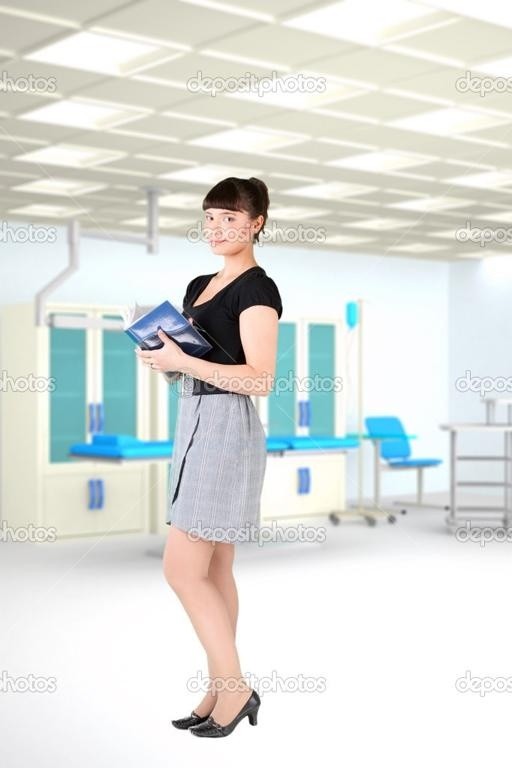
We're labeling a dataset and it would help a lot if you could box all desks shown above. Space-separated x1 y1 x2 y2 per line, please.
345 431 422 515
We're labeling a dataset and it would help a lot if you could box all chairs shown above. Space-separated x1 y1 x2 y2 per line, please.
362 415 450 513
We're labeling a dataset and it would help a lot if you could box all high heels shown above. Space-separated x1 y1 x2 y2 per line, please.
188 685 262 739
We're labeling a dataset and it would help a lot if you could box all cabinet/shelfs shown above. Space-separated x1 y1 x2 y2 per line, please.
1 304 359 543
439 398 512 532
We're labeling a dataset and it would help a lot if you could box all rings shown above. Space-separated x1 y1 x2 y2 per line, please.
150 363 155 370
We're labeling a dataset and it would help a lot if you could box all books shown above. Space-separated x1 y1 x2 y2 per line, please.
123 297 213 385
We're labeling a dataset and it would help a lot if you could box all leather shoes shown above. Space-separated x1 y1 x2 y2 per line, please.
170 709 214 731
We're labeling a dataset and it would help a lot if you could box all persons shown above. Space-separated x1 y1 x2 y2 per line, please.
134 177 283 737
